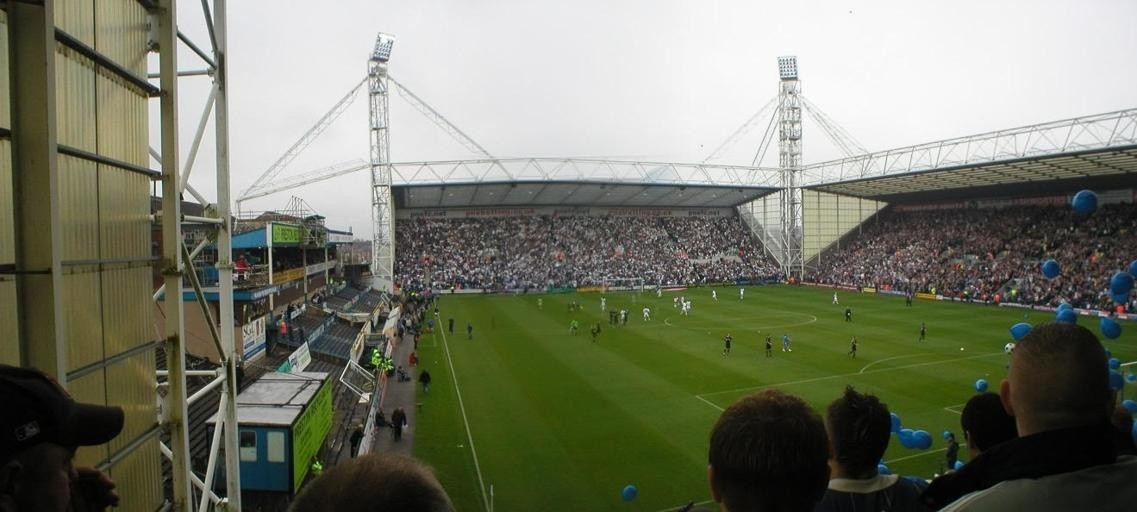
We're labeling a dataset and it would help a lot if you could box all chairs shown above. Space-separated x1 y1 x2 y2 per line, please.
937 452 1137 512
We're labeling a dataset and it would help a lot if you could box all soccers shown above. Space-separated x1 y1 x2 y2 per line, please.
1003 342 1015 352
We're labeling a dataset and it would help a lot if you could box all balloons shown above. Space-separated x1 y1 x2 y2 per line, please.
1073 191 1099 220
877 464 890 475
943 431 950 439
1011 260 1135 414
975 379 987 392
889 412 931 449
954 460 964 470
623 485 637 500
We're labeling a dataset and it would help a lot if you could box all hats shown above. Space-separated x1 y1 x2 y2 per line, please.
2 363 125 450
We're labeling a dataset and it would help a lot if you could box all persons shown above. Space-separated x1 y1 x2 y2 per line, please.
764 305 929 359
236 362 244 394
707 389 831 511
288 450 457 510
932 322 1134 511
349 406 407 458
393 215 782 295
826 387 930 511
236 255 248 288
369 290 438 393
280 276 346 344
0 362 124 510
310 455 323 477
448 315 455 335
536 287 744 359
467 323 472 339
805 202 1136 316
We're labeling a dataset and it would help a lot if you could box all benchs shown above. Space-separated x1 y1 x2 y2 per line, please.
278 284 386 364
